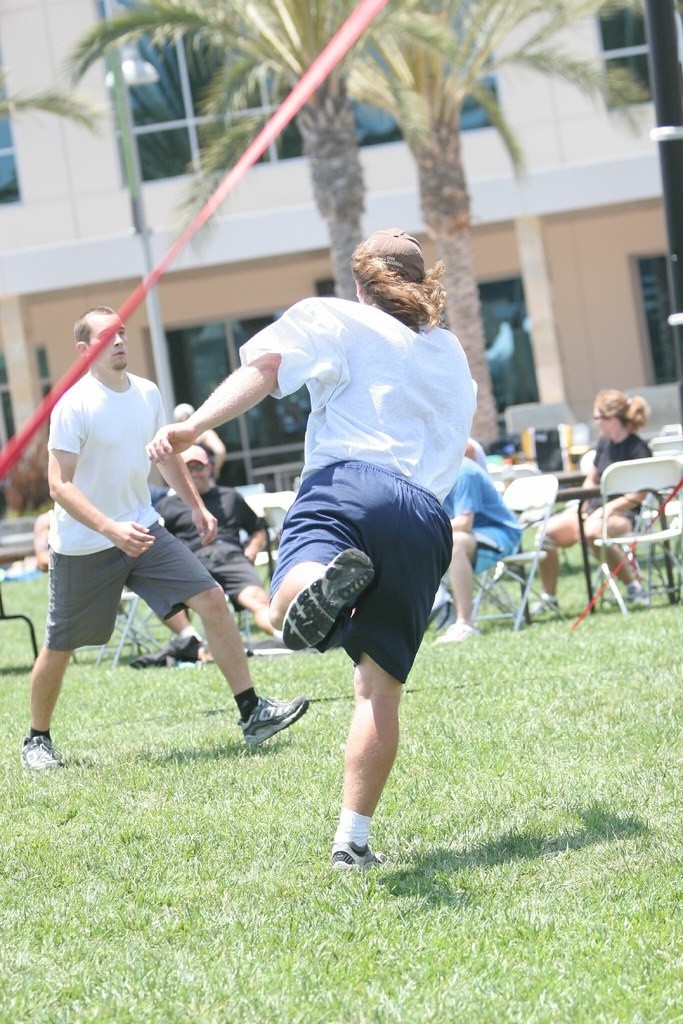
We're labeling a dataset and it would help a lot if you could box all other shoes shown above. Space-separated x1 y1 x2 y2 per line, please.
528 592 560 616
433 622 483 645
627 580 648 607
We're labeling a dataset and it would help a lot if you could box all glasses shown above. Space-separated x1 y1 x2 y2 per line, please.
188 466 204 472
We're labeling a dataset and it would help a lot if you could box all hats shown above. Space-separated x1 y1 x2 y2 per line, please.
180 445 208 465
364 228 425 284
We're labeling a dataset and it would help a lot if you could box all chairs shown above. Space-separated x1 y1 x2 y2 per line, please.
470 424 682 632
97 489 300 666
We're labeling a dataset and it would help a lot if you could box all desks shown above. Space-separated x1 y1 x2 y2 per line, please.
519 487 676 622
0 548 38 660
544 470 588 483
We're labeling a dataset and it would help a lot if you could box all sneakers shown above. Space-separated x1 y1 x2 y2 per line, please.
282 547 375 650
21 736 68 778
238 696 309 746
330 840 390 871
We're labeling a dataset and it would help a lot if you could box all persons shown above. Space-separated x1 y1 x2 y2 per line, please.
144 226 479 874
526 389 655 616
21 304 311 777
432 436 522 646
159 402 284 663
33 508 55 574
